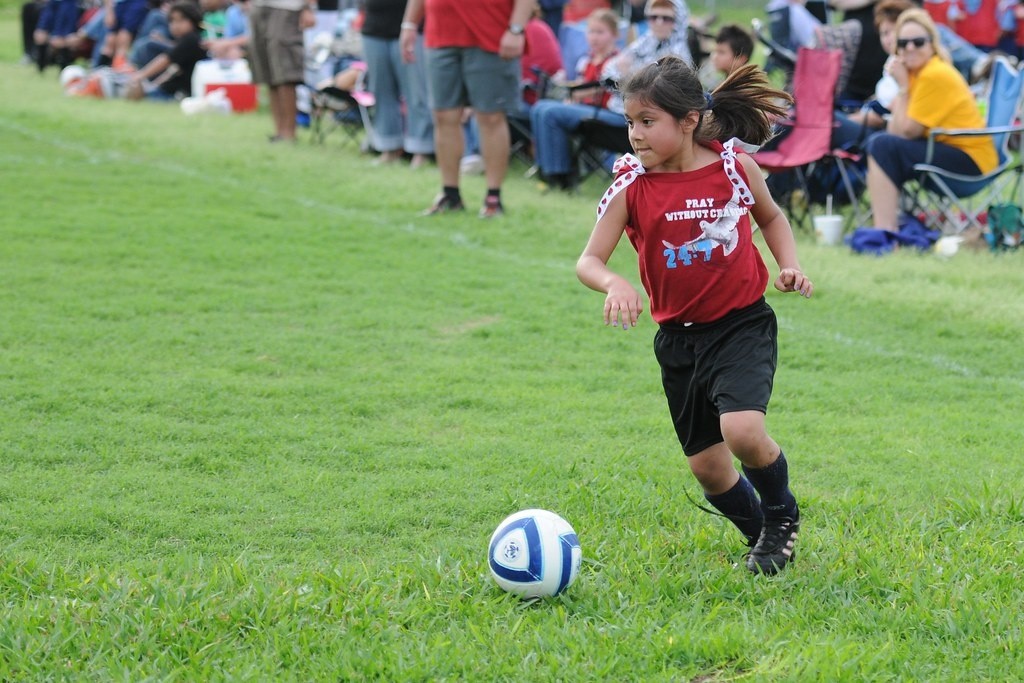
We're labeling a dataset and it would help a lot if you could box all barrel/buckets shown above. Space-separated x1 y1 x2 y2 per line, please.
813 214 843 245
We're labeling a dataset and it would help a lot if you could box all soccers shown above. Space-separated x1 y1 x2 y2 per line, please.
488 510 580 601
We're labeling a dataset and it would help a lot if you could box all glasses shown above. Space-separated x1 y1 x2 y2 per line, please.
645 14 675 26
897 37 933 49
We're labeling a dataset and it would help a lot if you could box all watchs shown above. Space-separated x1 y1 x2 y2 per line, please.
506 23 525 36
304 3 319 11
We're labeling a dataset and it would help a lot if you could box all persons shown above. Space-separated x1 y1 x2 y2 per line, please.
525 0 1024 233
398 1 538 219
17 1 364 121
575 57 815 574
239 1 319 150
361 0 434 168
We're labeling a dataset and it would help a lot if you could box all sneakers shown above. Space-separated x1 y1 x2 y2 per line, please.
740 503 800 575
417 195 464 219
479 197 504 221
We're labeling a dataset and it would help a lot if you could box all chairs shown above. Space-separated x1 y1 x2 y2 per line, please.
297 55 1023 247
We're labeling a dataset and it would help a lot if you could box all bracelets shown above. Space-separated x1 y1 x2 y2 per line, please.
400 22 420 30
